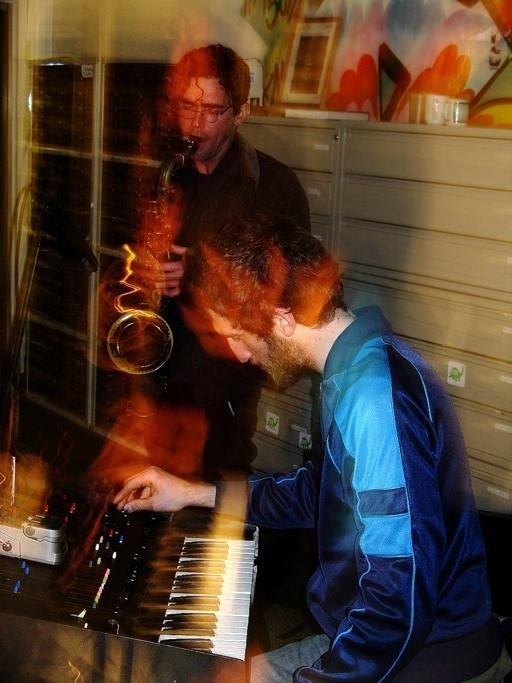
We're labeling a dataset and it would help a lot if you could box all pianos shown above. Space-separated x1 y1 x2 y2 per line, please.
116 504 264 662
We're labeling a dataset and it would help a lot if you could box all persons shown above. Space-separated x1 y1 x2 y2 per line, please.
115 207 490 681
106 37 309 477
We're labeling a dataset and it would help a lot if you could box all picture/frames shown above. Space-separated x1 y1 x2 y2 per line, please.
278 17 342 109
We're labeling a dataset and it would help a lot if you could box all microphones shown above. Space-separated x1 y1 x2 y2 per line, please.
32 186 99 274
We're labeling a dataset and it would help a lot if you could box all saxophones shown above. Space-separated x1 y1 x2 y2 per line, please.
106 135 198 371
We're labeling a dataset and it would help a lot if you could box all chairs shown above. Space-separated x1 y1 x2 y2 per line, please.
476 511 512 621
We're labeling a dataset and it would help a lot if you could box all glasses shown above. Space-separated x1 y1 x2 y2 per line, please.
179 103 233 123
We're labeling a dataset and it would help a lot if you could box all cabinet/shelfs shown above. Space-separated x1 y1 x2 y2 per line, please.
3 54 512 519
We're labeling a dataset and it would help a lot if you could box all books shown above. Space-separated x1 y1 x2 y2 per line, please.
285 106 368 120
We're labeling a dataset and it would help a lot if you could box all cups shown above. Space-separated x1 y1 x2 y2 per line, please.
409 94 470 126
